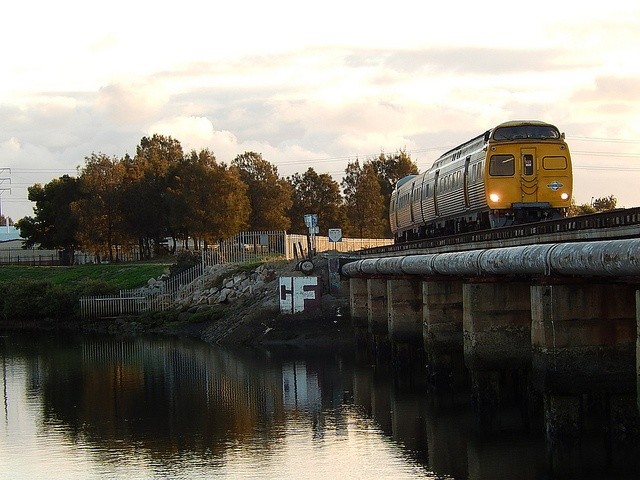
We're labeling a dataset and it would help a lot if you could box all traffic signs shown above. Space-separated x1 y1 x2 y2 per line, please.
304 215 317 227
329 229 342 242
309 227 319 234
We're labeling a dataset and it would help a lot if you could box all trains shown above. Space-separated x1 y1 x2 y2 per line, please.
389 120 573 244
